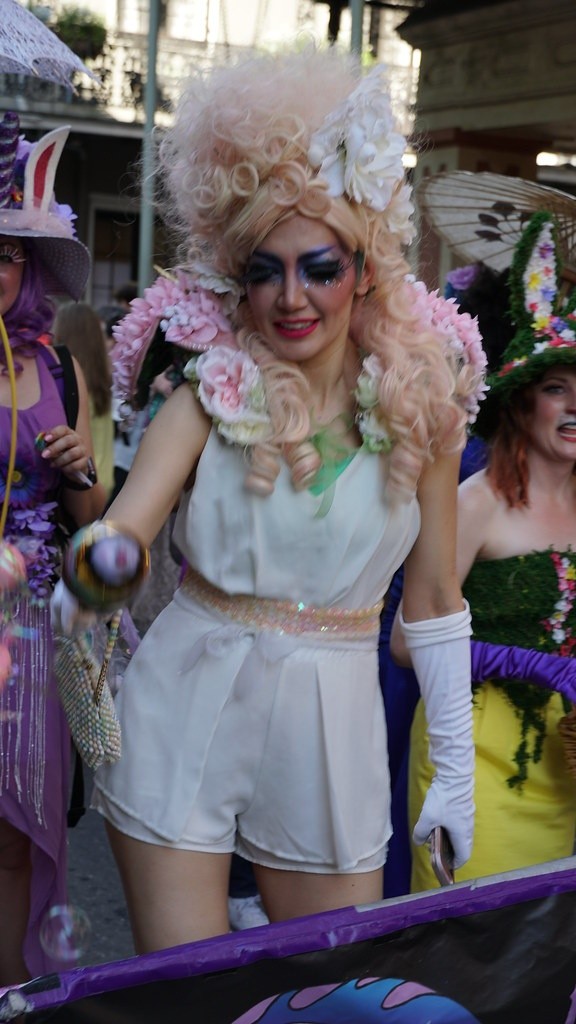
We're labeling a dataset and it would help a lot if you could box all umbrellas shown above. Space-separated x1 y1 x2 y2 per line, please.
414 169 576 293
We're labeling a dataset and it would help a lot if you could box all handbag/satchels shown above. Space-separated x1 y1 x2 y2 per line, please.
54 610 122 772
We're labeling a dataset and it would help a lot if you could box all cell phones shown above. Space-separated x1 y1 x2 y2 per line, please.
429 825 454 888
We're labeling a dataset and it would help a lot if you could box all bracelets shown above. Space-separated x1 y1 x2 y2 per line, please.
66 457 97 490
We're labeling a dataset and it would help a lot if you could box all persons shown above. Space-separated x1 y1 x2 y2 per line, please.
0 112 107 989
53 280 173 508
391 319 576 893
50 51 476 957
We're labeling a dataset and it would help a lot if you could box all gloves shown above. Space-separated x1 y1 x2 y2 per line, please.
398 597 474 870
468 640 576 703
49 578 98 637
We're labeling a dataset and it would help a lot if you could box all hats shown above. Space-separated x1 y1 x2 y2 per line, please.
469 212 576 444
0 111 91 304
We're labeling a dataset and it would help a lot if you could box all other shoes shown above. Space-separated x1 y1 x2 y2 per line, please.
227 893 271 932
